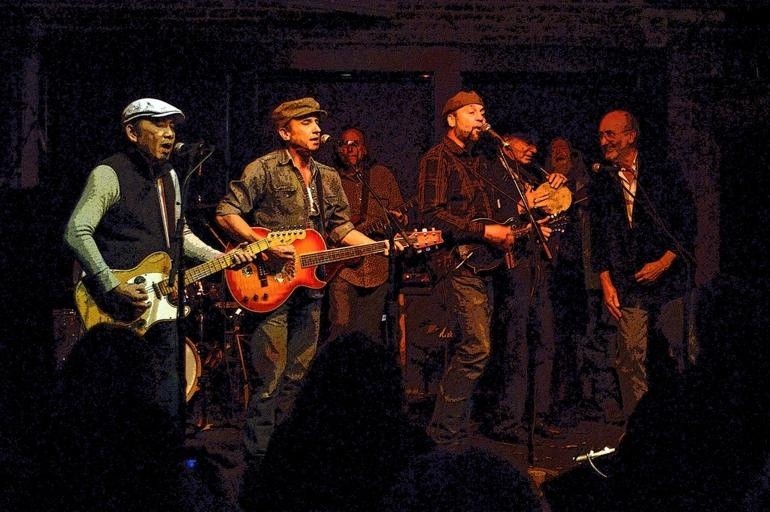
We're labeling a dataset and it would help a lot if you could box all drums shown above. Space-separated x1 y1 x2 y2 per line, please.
54 309 82 366
536 180 572 214
185 337 203 404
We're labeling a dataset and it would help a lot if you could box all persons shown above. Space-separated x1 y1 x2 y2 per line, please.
416 91 554 453
585 110 698 444
61 97 257 441
215 97 408 473
318 124 706 460
0 286 762 511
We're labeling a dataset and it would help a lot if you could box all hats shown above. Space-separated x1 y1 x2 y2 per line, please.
122 98 186 124
272 98 328 130
440 91 486 128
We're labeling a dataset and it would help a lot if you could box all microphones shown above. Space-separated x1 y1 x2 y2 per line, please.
592 163 629 173
320 134 357 148
481 123 513 152
173 142 215 155
530 160 550 177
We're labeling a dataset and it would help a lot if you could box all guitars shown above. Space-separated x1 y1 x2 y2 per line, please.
459 211 567 273
74 224 305 337
315 193 417 283
225 228 444 314
